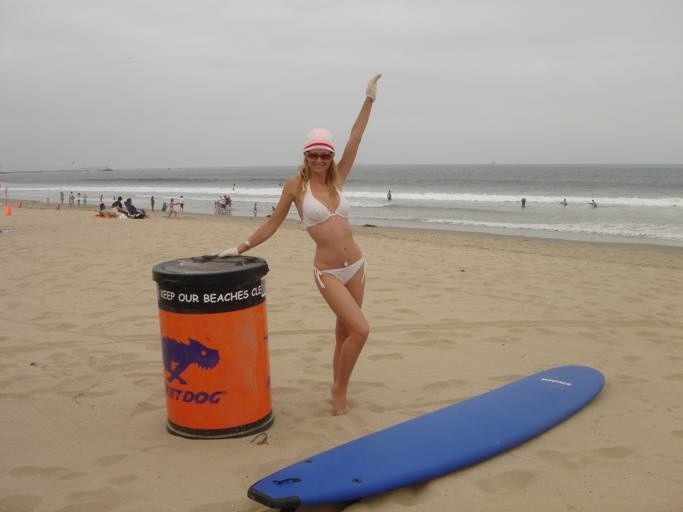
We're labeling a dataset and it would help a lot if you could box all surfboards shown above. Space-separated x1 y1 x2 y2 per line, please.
248 365 605 511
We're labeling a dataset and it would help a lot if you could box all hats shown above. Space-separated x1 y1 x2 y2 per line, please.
303 128 336 152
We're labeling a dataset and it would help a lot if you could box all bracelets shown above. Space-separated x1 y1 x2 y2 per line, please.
242 238 252 250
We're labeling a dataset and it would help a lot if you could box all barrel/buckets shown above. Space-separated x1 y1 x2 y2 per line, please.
150 253 275 439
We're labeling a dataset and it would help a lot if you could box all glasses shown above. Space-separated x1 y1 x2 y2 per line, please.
309 153 334 160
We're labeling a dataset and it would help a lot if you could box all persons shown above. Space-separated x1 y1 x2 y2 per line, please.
560 198 568 207
233 183 235 190
253 201 257 217
387 189 391 200
271 206 275 212
60 191 233 218
522 198 526 208
588 200 598 208
209 67 384 416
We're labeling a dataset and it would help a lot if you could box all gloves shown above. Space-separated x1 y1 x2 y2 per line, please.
366 73 382 102
206 248 240 257
